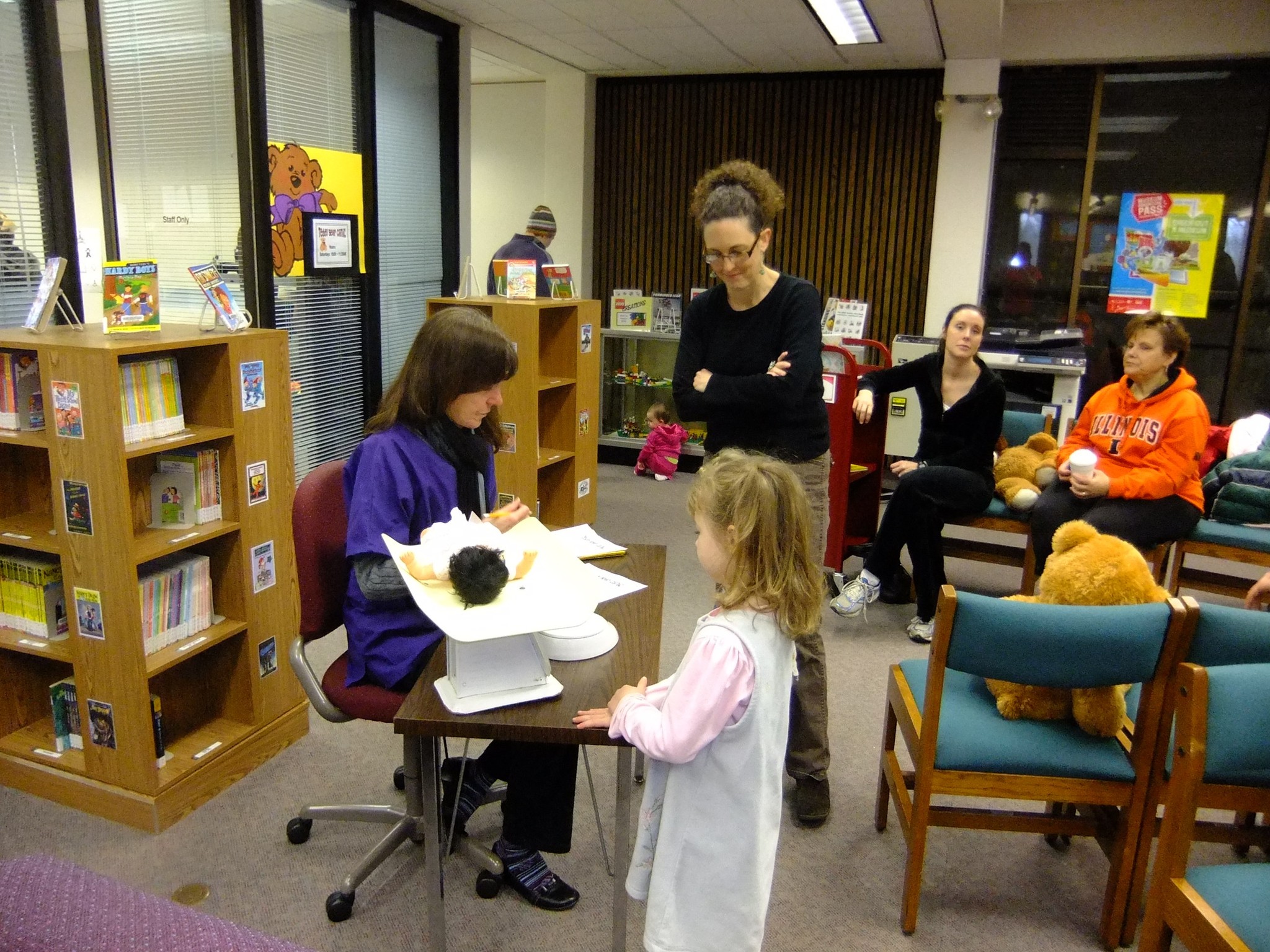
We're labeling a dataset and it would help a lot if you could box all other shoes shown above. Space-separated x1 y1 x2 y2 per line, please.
794 775 829 821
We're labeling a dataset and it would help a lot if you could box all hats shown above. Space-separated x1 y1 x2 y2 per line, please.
526 205 557 237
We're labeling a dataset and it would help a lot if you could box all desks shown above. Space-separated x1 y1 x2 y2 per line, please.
392 545 675 952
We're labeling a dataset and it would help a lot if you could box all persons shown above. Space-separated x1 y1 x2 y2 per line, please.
1059 298 1093 345
1 211 44 294
1244 570 1270 612
672 157 840 824
485 203 560 299
1030 311 1206 567
632 401 688 480
996 241 1041 316
830 302 1006 645
568 442 822 951
337 304 594 910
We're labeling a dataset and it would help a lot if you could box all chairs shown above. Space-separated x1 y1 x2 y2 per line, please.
1140 662 1270 952
1121 596 1270 947
876 583 1186 950
908 411 1052 602
1051 418 1175 587
287 461 509 920
1168 425 1270 598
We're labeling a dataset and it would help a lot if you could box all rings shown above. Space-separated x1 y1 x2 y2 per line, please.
767 359 776 372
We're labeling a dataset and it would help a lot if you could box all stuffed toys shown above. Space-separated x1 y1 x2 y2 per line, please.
991 429 1065 507
980 515 1170 740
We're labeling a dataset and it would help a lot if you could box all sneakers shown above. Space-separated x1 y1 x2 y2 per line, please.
828 574 880 618
909 616 935 642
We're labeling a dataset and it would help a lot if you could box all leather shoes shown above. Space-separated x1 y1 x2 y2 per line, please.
491 840 580 911
441 757 488 854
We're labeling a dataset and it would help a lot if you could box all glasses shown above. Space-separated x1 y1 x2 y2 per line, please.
701 230 760 263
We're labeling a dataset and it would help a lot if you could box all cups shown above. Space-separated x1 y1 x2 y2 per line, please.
1070 449 1096 479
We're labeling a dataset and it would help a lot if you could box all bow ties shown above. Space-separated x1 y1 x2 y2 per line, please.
270 190 324 226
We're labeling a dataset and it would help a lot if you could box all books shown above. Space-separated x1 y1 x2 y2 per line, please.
492 259 507 297
540 263 578 300
24 256 69 334
507 259 536 299
102 258 163 335
188 263 249 333
0 345 230 763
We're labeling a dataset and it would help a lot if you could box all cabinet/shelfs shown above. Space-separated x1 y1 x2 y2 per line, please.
598 328 708 457
538 305 578 531
0 347 90 773
822 337 892 573
119 343 258 785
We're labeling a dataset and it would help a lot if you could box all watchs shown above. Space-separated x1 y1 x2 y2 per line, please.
917 461 926 470
